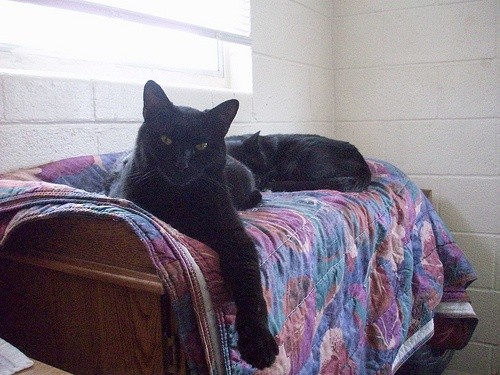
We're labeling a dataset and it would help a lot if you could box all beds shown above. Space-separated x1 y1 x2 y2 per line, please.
1 148 433 375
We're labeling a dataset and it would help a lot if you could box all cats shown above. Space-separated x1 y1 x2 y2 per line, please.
222 130 372 193
105 80 280 370
